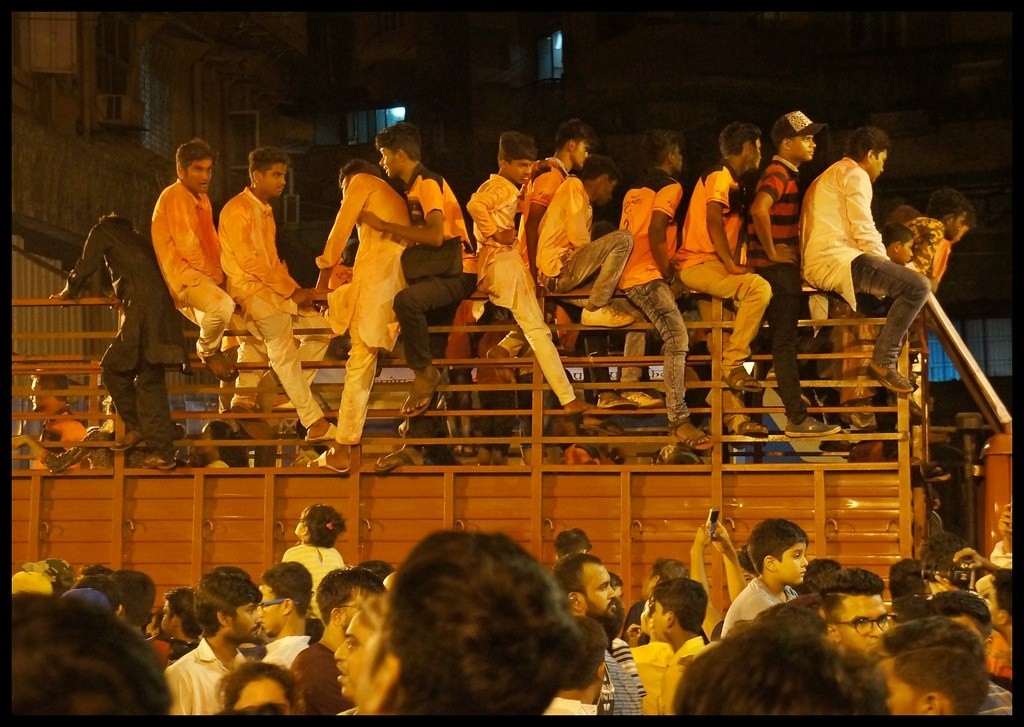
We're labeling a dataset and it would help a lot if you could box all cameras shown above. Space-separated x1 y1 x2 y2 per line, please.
921 558 972 592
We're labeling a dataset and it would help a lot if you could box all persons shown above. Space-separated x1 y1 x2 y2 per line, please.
690 518 749 643
720 517 809 643
49 110 977 469
990 499 1012 569
281 502 351 619
11 528 1012 716
29 369 91 471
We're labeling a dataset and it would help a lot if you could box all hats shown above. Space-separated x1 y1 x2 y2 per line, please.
554 527 593 559
21 558 75 588
12 570 53 596
769 110 831 148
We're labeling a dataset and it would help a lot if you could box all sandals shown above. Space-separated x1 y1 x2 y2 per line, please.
729 421 769 450
667 417 715 450
721 366 762 393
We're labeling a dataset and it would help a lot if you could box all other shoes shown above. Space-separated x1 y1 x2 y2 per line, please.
231 404 272 443
866 362 915 392
785 414 842 437
196 341 240 382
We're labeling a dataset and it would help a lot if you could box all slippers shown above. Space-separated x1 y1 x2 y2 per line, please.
374 449 416 473
306 450 351 473
304 422 337 443
579 419 629 438
271 399 296 412
399 370 441 418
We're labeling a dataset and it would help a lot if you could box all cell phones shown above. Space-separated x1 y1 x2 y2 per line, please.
707 510 719 534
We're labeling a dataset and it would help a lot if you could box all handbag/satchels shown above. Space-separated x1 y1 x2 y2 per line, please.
401 235 464 286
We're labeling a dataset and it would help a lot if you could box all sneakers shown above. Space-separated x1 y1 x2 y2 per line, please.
625 392 664 408
109 430 144 452
137 451 177 469
581 306 635 327
596 390 639 409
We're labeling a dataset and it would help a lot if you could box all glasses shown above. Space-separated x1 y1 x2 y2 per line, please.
256 598 300 614
825 614 896 635
